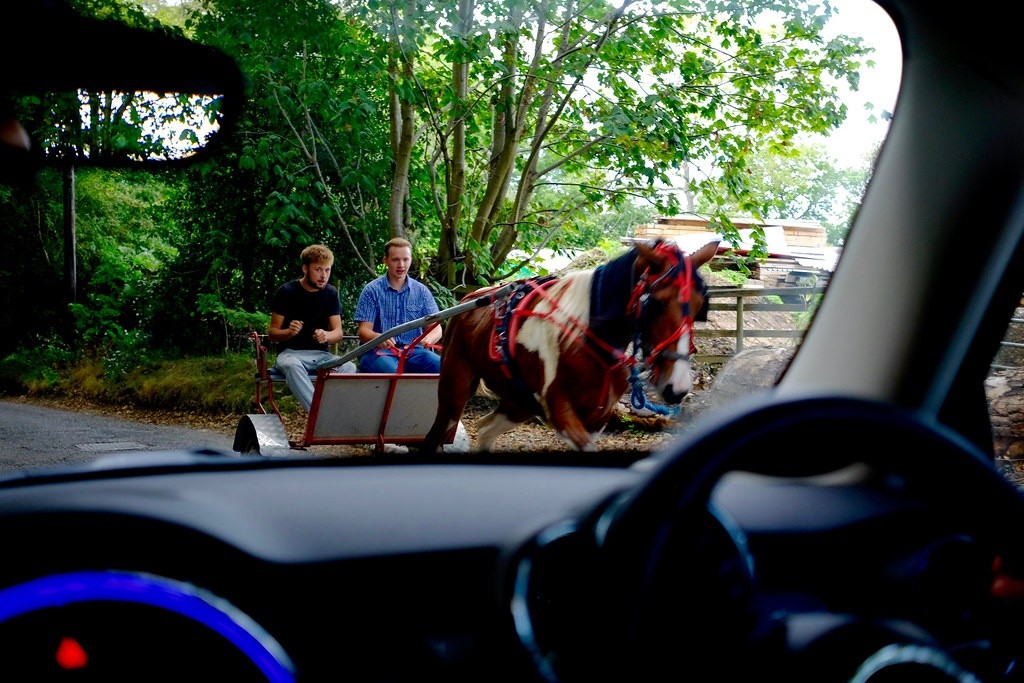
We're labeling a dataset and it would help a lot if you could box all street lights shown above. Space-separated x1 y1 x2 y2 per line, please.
0 112 76 307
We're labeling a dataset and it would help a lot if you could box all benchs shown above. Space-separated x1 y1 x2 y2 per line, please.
248 331 444 418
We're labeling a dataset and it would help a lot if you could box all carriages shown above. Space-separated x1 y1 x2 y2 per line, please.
231 236 720 457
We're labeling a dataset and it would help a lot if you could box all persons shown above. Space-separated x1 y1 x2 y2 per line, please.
268 244 357 415
354 237 442 374
0 105 30 156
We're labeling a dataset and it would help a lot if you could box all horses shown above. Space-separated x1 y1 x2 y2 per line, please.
417 235 722 450
